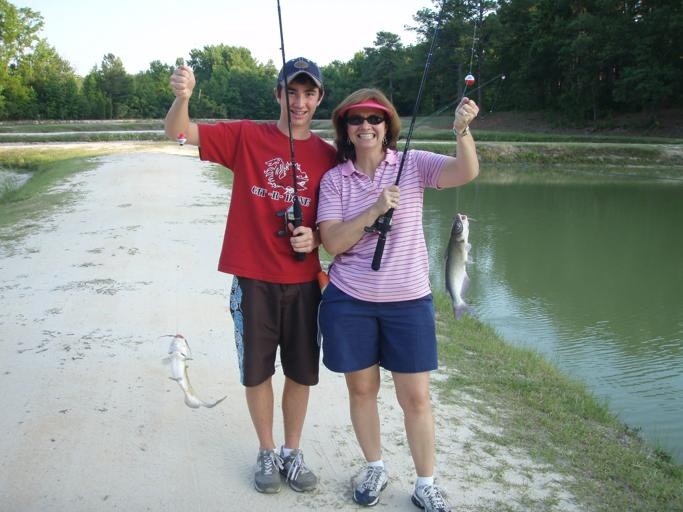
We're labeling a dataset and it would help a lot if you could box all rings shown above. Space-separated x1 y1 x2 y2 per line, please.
463 111 468 116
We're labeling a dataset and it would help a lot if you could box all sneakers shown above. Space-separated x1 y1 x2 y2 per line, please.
411 484 451 512
279 445 318 492
354 467 388 506
255 445 281 493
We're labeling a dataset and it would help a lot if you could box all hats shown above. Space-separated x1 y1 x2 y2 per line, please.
278 57 322 89
339 99 392 118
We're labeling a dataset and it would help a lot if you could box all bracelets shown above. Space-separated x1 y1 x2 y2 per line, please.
453 123 470 137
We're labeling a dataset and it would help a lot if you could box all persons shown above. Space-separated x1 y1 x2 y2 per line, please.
164 56 348 493
318 87 480 512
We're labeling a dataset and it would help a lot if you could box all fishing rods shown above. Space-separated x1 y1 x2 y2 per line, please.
371 1 451 270
276 1 307 261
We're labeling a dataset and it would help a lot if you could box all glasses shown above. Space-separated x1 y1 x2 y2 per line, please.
347 115 385 125
